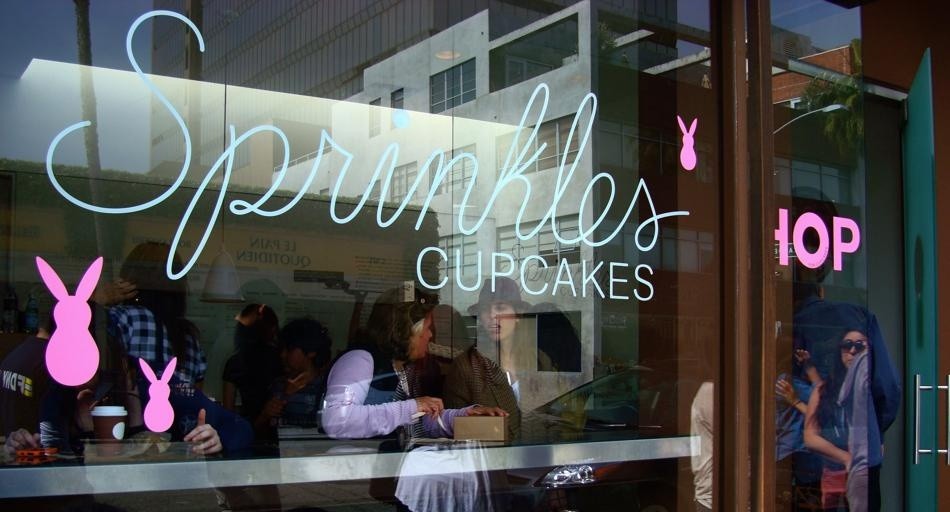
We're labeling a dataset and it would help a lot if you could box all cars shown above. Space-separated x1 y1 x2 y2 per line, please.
506 361 677 511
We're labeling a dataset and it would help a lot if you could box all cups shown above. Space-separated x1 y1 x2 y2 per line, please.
89 406 129 445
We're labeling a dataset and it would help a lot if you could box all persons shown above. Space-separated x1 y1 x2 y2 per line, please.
0 239 897 511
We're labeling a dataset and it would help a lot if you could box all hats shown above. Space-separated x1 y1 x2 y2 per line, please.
380 288 438 334
466 276 535 319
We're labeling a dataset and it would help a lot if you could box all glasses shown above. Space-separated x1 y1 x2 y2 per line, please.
839 339 870 354
